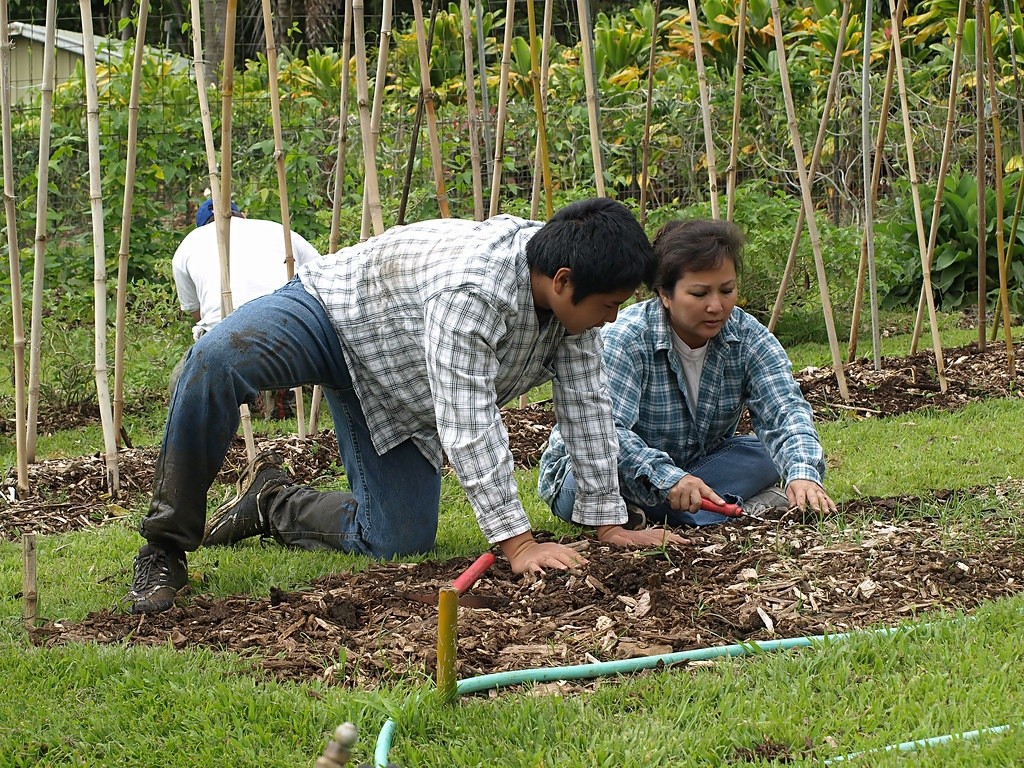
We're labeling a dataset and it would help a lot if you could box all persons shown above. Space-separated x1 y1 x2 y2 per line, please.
128 197 693 619
168 194 323 422
535 220 837 528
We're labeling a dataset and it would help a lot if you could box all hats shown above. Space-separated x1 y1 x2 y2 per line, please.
196 199 238 226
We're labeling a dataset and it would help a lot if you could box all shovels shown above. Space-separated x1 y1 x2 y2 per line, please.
403 551 500 611
700 498 802 527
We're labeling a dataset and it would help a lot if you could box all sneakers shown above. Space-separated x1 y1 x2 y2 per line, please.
127 546 188 613
201 452 289 549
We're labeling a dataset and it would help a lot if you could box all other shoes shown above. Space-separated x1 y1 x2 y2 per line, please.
265 389 295 421
622 503 646 531
739 486 809 523
